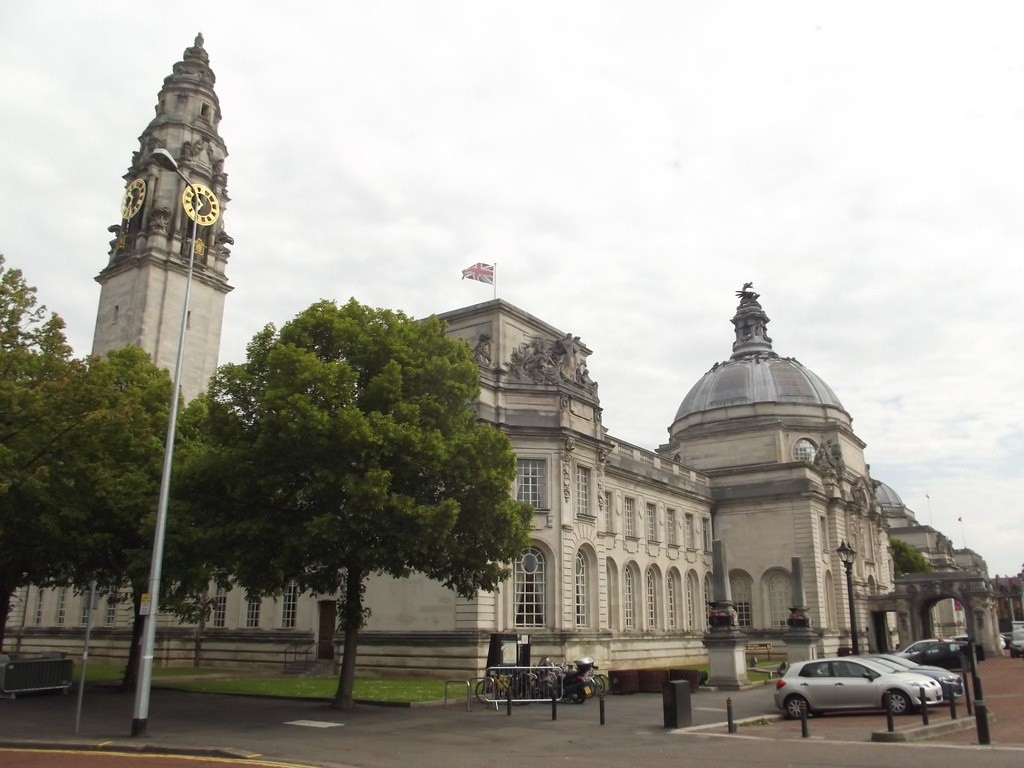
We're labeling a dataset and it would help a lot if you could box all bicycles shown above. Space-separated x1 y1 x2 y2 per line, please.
476 656 608 704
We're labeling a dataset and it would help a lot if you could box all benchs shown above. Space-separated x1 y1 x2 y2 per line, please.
3 656 75 699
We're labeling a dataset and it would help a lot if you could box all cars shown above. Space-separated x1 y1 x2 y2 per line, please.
774 655 944 720
816 654 964 700
892 620 1024 671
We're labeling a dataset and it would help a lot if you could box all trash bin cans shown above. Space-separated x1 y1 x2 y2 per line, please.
662 680 693 728
835 647 854 656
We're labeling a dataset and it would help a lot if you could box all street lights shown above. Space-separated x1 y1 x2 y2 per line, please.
835 538 859 655
130 146 199 735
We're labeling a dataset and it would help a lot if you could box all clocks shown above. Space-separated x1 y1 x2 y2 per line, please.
182 184 220 227
119 178 146 219
